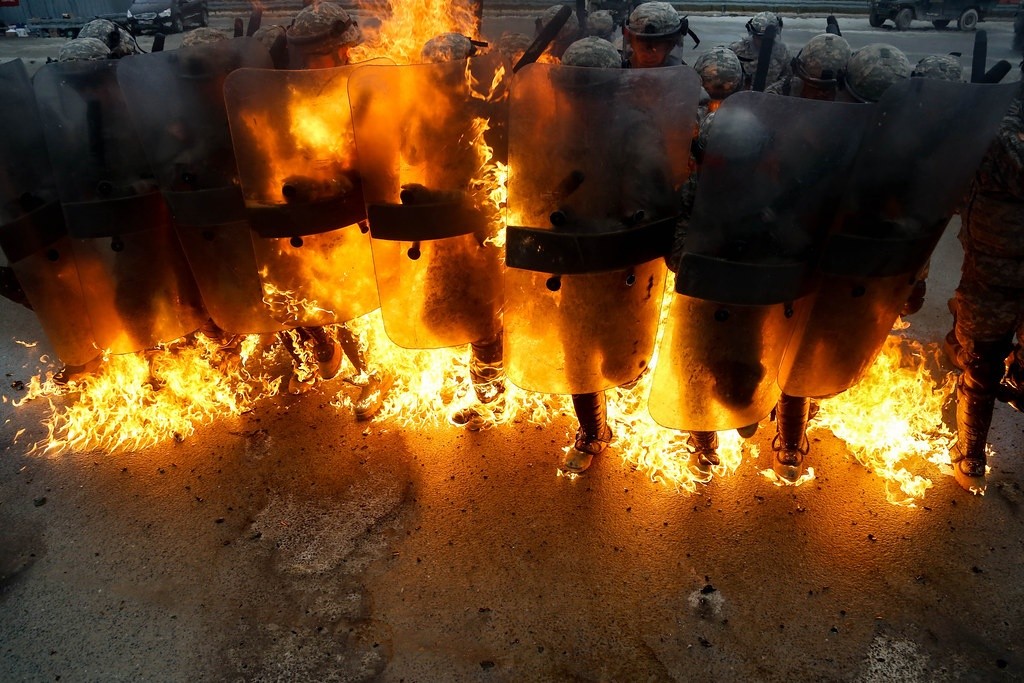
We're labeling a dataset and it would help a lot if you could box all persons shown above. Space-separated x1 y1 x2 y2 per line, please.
0 0 1024 494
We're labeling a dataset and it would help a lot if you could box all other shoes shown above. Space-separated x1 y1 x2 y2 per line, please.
772 432 811 486
288 369 315 395
313 337 343 379
945 331 971 373
565 424 594 473
686 437 720 484
947 431 986 493
353 369 393 421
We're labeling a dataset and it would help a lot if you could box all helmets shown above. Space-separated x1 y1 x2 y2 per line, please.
625 2 700 50
549 37 622 88
693 48 743 99
845 43 910 104
252 24 288 68
59 38 111 73
421 34 489 62
536 5 580 45
585 11 613 37
699 108 765 157
745 11 783 36
167 28 245 79
495 32 534 59
286 0 365 53
78 19 135 58
915 55 963 81
790 33 852 83
